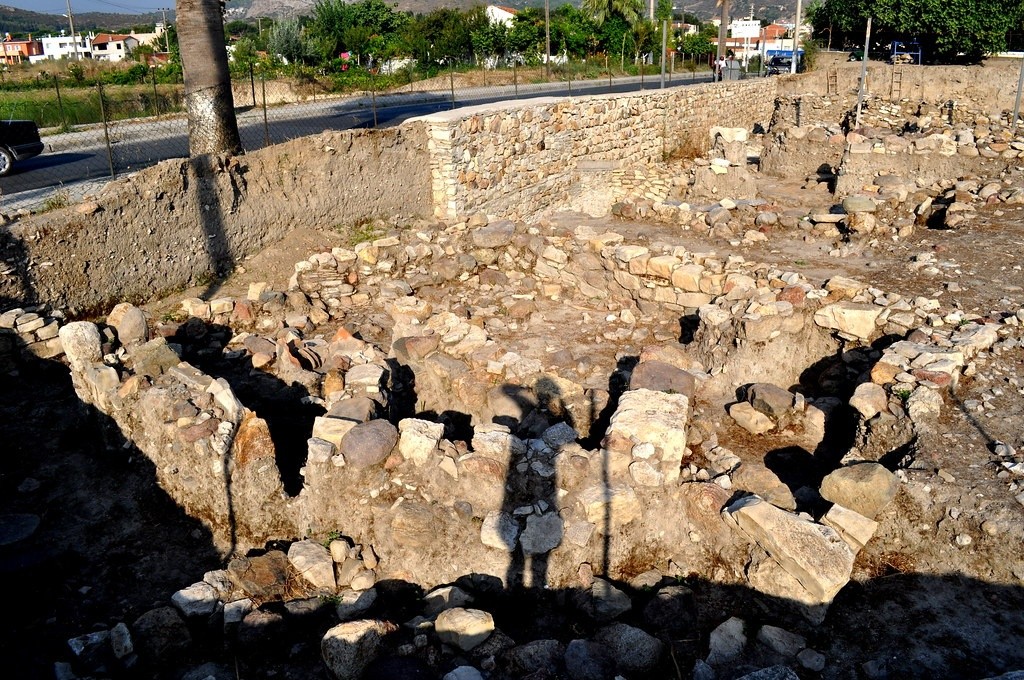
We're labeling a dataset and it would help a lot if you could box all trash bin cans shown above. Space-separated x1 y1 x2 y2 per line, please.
848 51 864 61
720 60 741 80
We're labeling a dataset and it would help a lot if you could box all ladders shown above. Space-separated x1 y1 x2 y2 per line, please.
890 57 904 102
826 67 838 94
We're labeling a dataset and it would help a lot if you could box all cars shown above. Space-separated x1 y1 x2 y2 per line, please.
765 56 799 77
0 120 44 176
